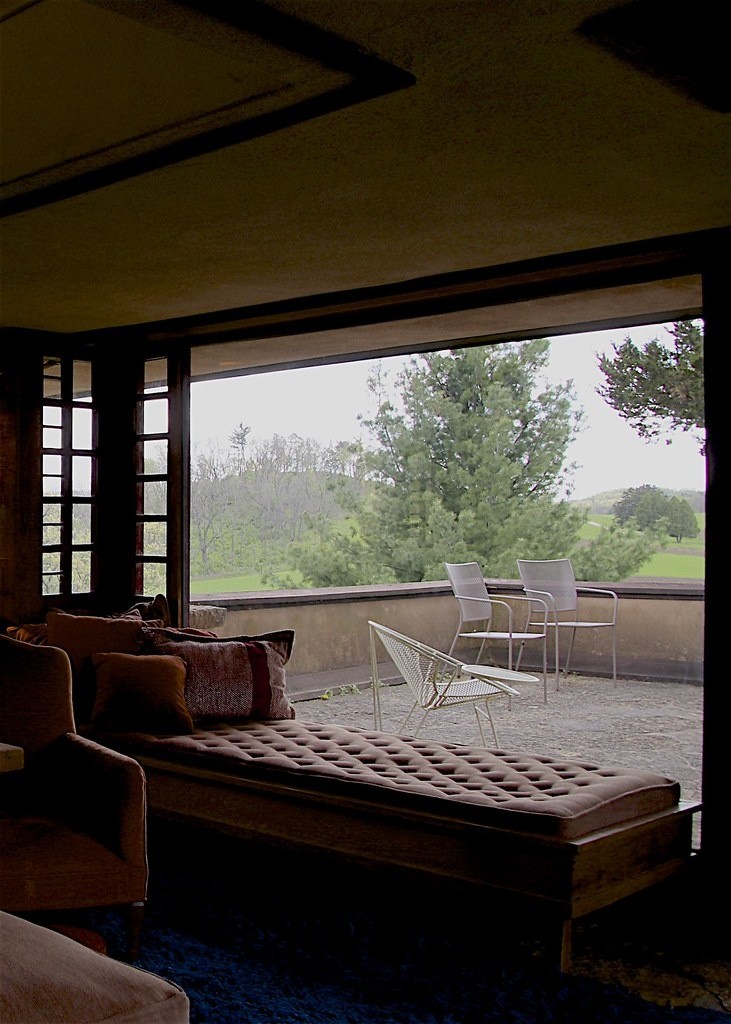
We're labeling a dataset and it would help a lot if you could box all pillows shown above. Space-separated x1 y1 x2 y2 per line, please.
8 594 171 644
47 608 161 708
91 648 196 735
139 625 296 722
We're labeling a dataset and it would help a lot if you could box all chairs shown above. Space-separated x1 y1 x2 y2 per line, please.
440 561 550 709
515 556 618 695
0 632 150 910
367 620 518 748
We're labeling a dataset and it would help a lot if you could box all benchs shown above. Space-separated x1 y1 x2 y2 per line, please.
8 595 703 975
